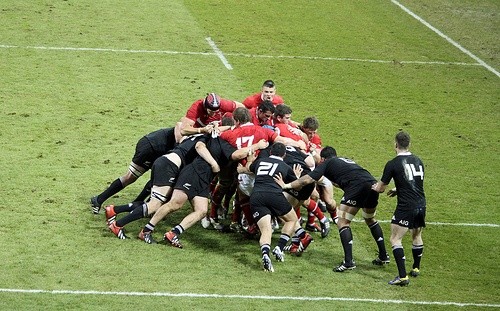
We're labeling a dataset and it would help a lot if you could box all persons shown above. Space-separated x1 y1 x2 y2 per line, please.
104 127 221 240
89 124 185 214
137 118 271 249
273 145 392 275
242 80 282 111
179 94 247 137
236 142 306 274
371 130 427 286
201 101 338 258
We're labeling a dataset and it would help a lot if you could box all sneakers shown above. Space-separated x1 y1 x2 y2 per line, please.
110 221 130 239
103 204 117 226
333 260 356 272
283 233 313 256
372 254 390 265
333 216 338 224
389 276 409 286
306 224 321 232
201 210 279 235
262 254 274 273
273 247 284 263
164 231 183 248
410 268 420 276
321 218 331 238
137 229 152 244
91 196 101 214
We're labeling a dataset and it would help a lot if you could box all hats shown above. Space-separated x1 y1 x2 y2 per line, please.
205 93 221 111
